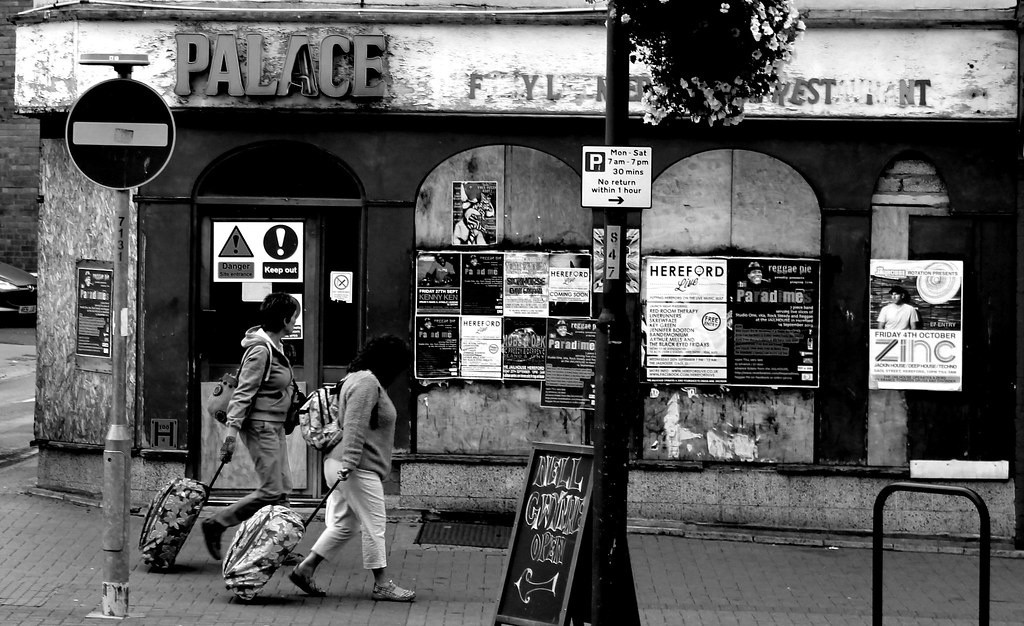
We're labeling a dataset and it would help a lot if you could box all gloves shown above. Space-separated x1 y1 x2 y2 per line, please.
219 436 236 463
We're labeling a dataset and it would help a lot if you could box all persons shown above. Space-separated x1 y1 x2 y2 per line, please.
876 286 919 331
287 333 417 600
425 256 456 285
201 293 300 561
550 321 573 339
744 261 770 287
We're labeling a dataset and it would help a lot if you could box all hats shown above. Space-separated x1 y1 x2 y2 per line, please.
746 262 764 273
555 320 568 328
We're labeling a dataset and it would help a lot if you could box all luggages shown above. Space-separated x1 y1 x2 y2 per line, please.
223 468 346 601
137 451 230 571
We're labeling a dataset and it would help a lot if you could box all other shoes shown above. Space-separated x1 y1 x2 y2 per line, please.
371 579 416 602
289 563 327 597
281 552 305 565
201 517 227 560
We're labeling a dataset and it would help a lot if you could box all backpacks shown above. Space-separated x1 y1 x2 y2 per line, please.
299 377 381 451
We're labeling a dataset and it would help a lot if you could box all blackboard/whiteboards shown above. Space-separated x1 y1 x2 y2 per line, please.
494 441 598 625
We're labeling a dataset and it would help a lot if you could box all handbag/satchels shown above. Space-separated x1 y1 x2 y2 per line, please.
284 389 306 435
208 343 272 422
